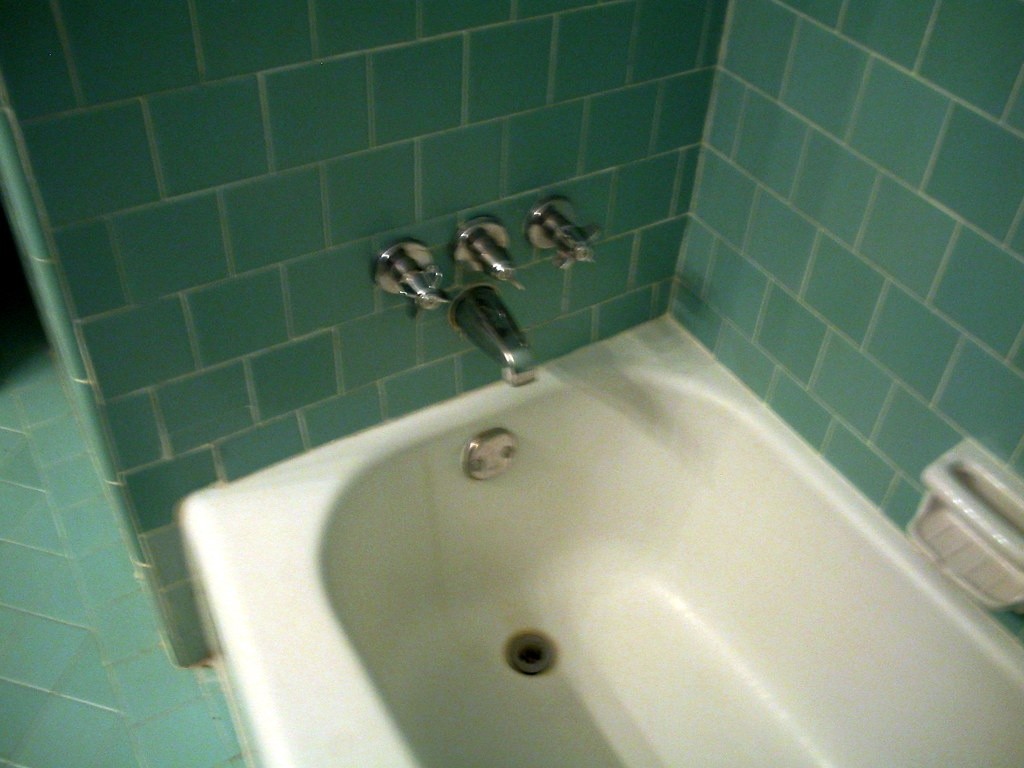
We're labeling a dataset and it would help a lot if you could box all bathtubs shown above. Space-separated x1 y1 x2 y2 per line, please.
181 315 1023 768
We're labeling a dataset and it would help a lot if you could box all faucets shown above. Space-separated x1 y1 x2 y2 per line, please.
451 286 533 388
